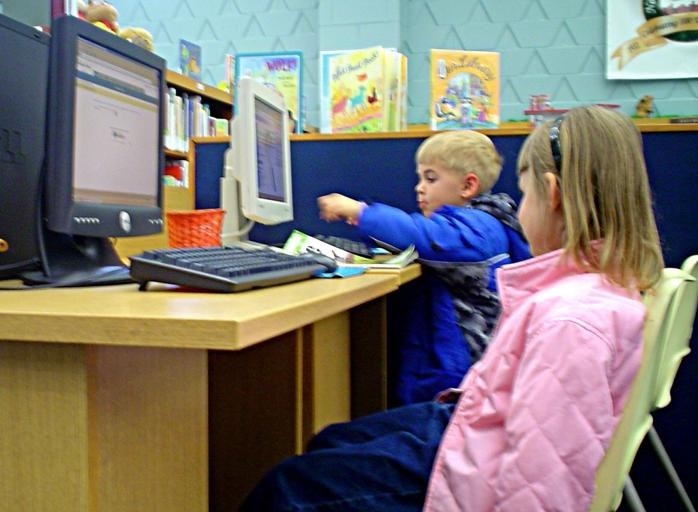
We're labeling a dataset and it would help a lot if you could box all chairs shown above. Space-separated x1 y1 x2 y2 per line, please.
589 256 698 512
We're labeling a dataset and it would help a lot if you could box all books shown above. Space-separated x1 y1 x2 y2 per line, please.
282 228 421 271
234 50 305 137
428 49 501 131
317 46 409 135
47 0 237 192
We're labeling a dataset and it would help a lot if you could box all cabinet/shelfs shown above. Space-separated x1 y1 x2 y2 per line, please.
108 69 232 262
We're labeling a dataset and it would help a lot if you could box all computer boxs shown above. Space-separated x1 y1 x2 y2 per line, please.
1 12 52 281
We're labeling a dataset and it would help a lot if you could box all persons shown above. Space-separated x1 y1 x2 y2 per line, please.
230 104 668 512
317 128 535 406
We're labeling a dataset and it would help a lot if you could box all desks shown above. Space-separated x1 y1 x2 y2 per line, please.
0 262 423 511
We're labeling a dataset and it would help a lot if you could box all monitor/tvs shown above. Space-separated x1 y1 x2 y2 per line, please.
221 76 294 254
24 13 168 285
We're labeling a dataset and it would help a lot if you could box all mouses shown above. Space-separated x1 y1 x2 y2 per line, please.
297 246 337 273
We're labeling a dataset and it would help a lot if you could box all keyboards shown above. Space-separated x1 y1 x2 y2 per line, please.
313 233 373 259
127 246 327 291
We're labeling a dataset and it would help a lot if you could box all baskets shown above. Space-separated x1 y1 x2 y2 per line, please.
166 208 227 247
